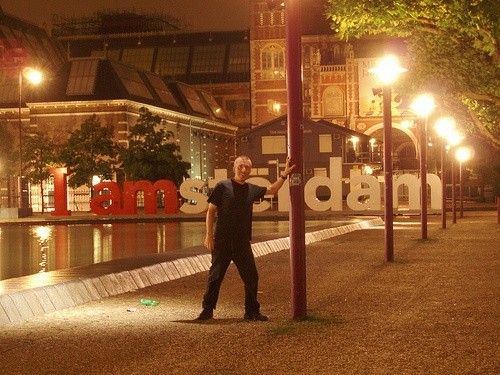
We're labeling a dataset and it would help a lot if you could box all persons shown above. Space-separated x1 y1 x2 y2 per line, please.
198 155 296 321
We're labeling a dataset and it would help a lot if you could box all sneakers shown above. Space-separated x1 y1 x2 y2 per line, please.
198 308 213 320
244 312 267 321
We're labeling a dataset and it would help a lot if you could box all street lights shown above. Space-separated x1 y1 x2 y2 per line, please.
18 67 39 211
449 133 456 224
459 148 464 219
379 59 393 261
438 120 447 227
417 99 427 239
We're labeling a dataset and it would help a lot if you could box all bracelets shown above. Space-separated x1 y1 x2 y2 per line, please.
281 175 287 179
